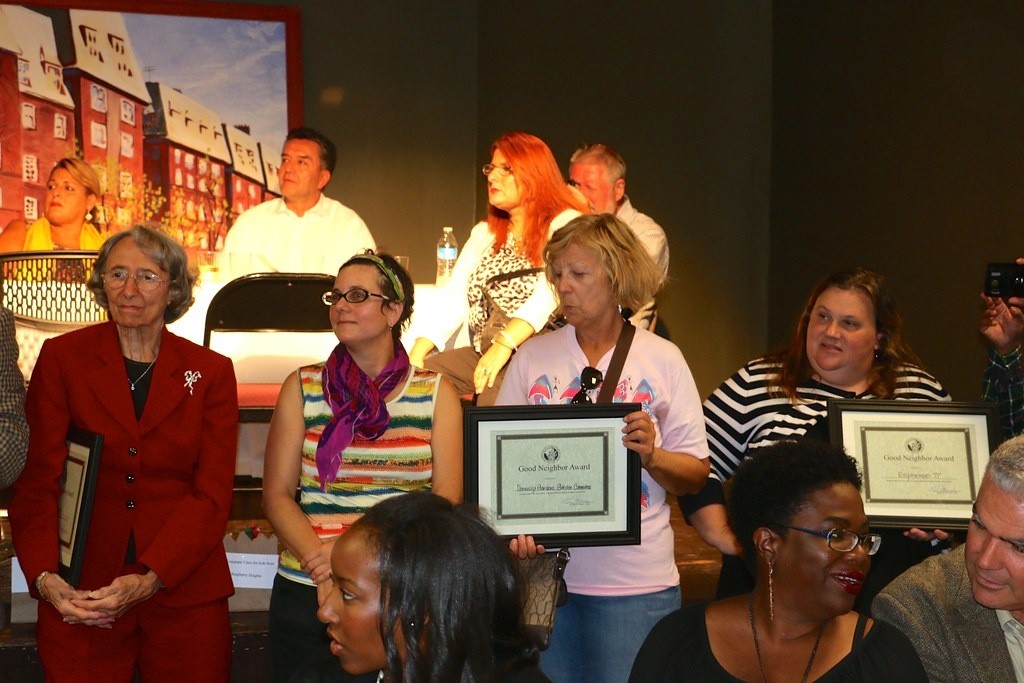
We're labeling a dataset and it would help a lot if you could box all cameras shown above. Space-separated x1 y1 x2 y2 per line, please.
984 263 1024 298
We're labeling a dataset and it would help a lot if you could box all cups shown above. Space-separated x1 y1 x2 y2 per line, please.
393 256 410 273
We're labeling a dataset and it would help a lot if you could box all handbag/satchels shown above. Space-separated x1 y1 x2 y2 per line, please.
481 306 551 357
516 551 570 652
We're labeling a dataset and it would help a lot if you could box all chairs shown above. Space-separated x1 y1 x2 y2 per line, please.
203 267 338 521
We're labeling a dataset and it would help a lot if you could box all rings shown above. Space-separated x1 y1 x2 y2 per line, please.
482 368 490 375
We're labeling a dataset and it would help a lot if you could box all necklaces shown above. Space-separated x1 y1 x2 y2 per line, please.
749 597 825 683
127 355 158 391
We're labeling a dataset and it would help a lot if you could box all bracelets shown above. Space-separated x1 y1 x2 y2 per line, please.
35 571 48 602
491 330 518 352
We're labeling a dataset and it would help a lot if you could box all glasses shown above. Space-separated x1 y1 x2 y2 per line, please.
482 162 513 175
321 289 392 303
780 520 882 559
99 269 171 293
572 367 603 407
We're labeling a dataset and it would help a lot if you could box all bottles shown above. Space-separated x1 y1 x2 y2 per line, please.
436 227 458 280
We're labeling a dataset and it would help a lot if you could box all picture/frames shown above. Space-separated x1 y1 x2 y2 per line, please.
827 395 999 529
57 427 106 591
459 402 642 547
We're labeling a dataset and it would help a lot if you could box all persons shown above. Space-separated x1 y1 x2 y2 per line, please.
872 436 1024 683
222 127 377 272
496 216 711 683
680 265 956 595
410 131 591 395
627 440 927 683
7 226 238 683
0 304 30 489
263 248 462 683
317 492 557 683
0 157 106 282
976 258 1024 444
567 142 670 329
